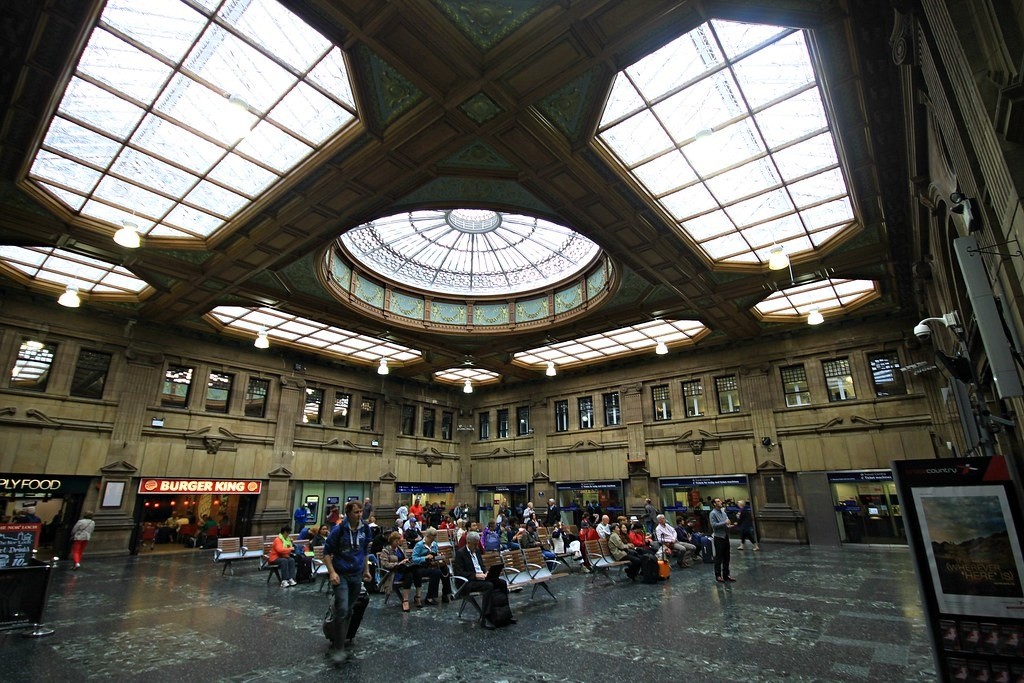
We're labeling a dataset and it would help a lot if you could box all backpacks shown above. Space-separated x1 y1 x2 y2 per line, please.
482 588 511 627
486 533 500 551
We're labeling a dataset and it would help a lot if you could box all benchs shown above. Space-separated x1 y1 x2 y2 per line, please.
214 523 704 618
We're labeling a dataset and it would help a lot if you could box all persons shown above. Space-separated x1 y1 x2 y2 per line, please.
709 497 738 582
294 503 307 534
363 498 372 520
326 505 339 526
368 517 378 527
164 504 228 549
26 507 63 546
734 500 759 551
362 496 715 629
309 524 330 551
336 514 344 525
322 502 372 662
298 525 319 540
71 510 95 570
268 524 297 587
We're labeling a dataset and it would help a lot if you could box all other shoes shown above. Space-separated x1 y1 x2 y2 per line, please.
484 617 496 630
332 647 347 665
573 556 582 561
442 595 449 602
625 568 635 581
678 555 714 568
72 563 80 571
737 547 743 550
425 598 438 605
288 578 297 586
717 577 725 583
281 580 289 588
727 576 736 582
414 596 423 608
509 618 518 623
752 547 759 551
581 566 590 573
402 600 410 612
325 648 354 659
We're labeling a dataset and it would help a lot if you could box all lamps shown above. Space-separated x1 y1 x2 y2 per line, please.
56 278 80 308
762 437 770 445
656 322 670 354
463 371 472 393
807 294 825 325
947 191 979 231
114 205 143 249
769 236 791 271
546 338 557 376
152 417 166 427
934 349 972 383
371 440 379 448
378 344 390 375
254 311 269 347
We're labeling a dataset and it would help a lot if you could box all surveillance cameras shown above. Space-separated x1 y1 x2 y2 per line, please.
914 324 931 339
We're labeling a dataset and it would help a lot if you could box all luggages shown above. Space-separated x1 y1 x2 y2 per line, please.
323 578 373 645
657 533 671 580
641 531 659 584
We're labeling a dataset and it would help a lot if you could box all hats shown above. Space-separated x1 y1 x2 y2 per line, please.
528 521 537 528
410 517 417 523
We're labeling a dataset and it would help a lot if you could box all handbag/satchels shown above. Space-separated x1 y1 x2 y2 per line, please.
428 559 450 578
